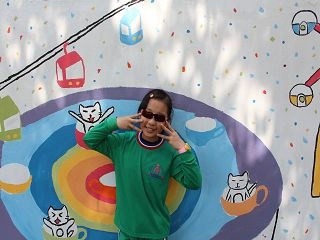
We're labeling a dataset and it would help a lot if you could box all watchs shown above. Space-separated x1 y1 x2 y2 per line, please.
178 142 191 154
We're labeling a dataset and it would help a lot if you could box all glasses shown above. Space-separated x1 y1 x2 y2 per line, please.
142 110 165 122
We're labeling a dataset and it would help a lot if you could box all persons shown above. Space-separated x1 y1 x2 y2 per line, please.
83 89 201 240
